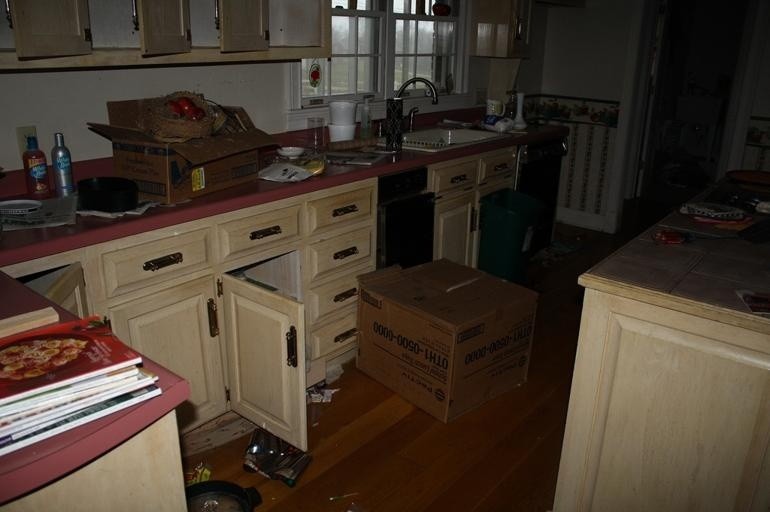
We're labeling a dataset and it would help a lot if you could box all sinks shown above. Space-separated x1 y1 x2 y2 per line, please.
373 123 513 153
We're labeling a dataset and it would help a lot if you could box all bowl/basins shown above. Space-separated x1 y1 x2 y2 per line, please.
78 176 138 214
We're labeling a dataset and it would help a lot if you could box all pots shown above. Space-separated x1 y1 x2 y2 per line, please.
184 480 263 511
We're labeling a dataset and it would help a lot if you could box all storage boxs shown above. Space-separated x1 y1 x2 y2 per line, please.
87 95 282 204
354 258 541 427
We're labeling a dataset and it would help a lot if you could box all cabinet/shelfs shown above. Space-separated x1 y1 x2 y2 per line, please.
0 1 331 70
3 177 380 451
424 147 520 268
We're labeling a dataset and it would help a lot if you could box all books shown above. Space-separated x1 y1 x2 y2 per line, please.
0 316 162 456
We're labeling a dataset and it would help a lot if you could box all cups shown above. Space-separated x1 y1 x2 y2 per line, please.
308 116 325 159
487 99 506 117
444 128 456 146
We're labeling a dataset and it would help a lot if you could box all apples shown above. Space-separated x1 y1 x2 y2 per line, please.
178 97 193 113
186 108 205 121
165 99 186 118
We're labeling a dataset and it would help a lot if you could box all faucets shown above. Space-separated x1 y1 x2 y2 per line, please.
394 77 439 104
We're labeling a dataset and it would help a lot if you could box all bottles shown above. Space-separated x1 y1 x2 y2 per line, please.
507 91 518 119
23 133 72 199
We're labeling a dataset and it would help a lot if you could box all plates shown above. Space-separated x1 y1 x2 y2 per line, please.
277 146 306 157
